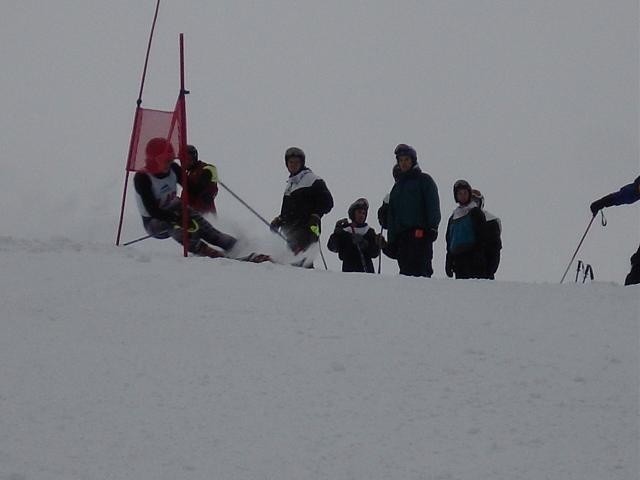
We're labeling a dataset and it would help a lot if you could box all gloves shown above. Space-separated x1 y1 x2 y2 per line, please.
307 214 321 234
270 218 284 231
352 233 363 244
591 198 604 217
445 254 453 277
334 218 347 234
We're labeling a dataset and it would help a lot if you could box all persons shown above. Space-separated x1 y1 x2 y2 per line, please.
469 189 505 280
377 143 440 279
327 196 394 274
445 178 489 280
132 135 273 262
589 170 640 285
270 147 333 267
177 146 219 216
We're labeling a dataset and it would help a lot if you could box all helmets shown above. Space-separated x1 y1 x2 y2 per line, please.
393 165 401 177
179 145 197 162
348 198 369 217
394 144 416 161
453 180 484 211
146 138 176 162
285 148 305 168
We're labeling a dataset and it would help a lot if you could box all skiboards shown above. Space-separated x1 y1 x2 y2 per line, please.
230 252 269 262
